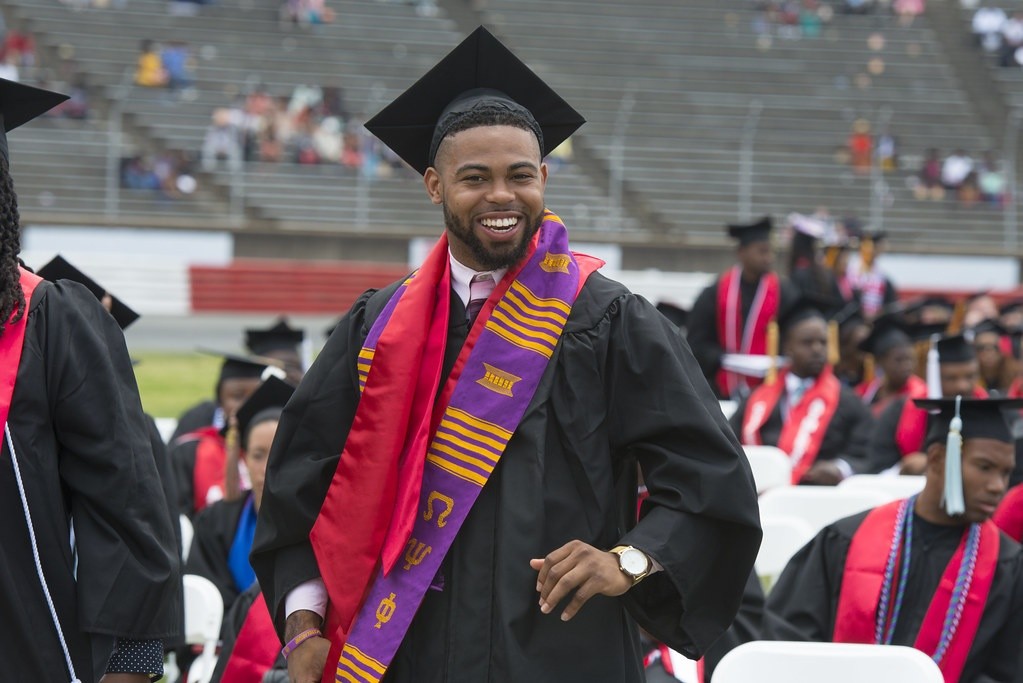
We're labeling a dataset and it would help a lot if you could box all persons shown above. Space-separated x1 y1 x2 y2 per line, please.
960 0 1023 67
691 209 1023 683
0 0 411 195
29 254 343 683
846 116 1012 206
755 0 926 41
249 24 762 683
0 79 186 683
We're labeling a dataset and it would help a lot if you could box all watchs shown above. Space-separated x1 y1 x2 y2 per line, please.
608 545 653 585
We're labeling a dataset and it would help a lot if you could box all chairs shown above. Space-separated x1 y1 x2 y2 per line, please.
183 574 225 683
835 474 927 513
751 485 870 583
740 445 791 493
710 640 944 683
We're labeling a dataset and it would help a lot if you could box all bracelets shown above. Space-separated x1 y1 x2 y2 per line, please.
281 628 323 660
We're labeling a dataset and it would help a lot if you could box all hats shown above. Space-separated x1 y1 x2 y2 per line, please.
209 321 307 428
908 398 1023 517
690 207 1022 415
362 24 588 173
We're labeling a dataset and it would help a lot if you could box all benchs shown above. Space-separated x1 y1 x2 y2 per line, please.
0 0 1023 259
